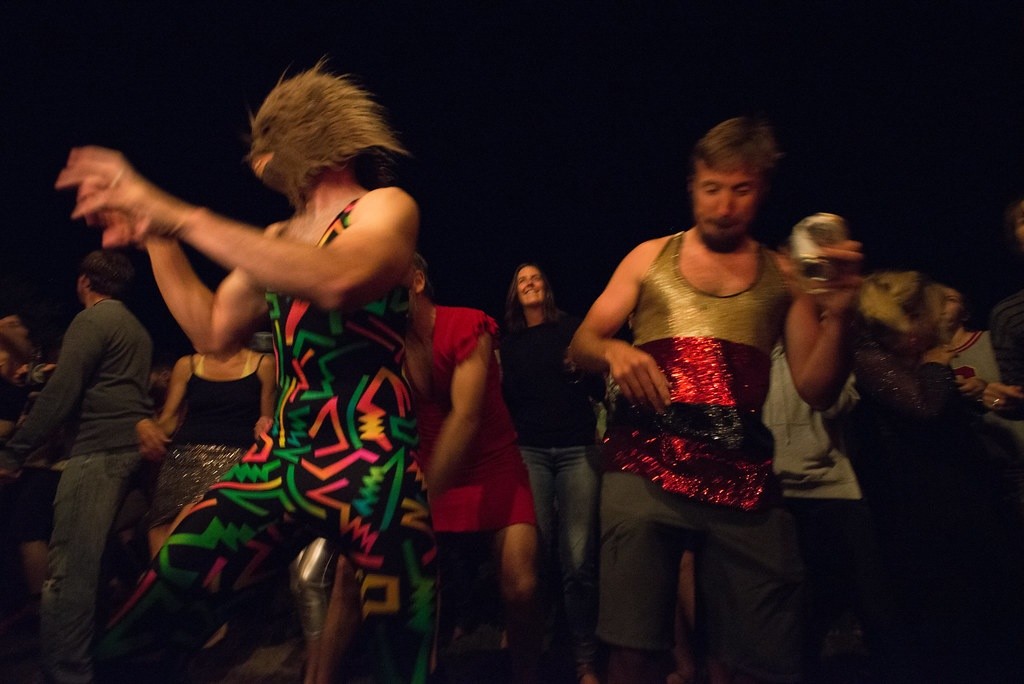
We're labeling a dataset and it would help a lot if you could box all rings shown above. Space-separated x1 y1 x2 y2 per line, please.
992 398 999 403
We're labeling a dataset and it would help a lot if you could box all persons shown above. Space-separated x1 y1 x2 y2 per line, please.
570 118 865 684
763 336 891 683
1 250 158 684
0 314 171 608
58 54 440 684
982 198 1023 420
853 266 960 428
497 264 608 684
289 538 337 683
135 345 277 561
928 266 1000 421
665 548 698 682
403 253 539 684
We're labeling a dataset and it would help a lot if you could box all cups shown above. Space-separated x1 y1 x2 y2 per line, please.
27 353 47 398
